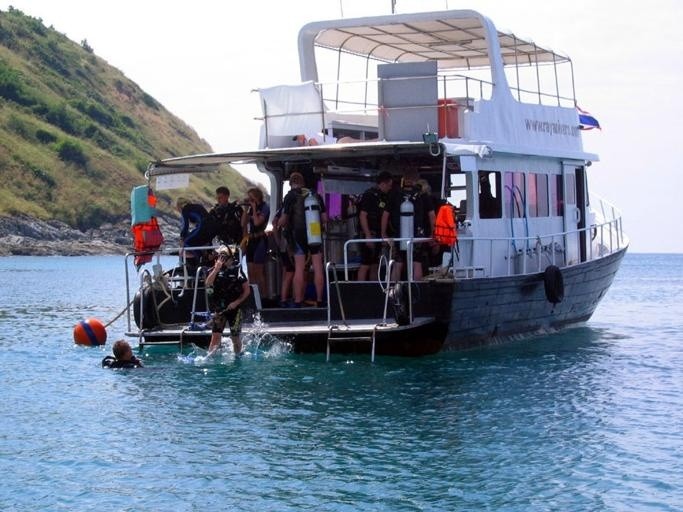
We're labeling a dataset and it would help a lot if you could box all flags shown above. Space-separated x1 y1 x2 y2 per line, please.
576 106 601 130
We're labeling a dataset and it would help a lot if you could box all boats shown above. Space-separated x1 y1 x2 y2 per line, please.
122 5 629 364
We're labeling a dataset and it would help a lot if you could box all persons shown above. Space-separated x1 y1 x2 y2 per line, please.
177 171 448 359
291 132 379 147
108 339 142 369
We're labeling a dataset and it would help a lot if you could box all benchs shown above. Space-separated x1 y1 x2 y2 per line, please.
327 110 378 142
